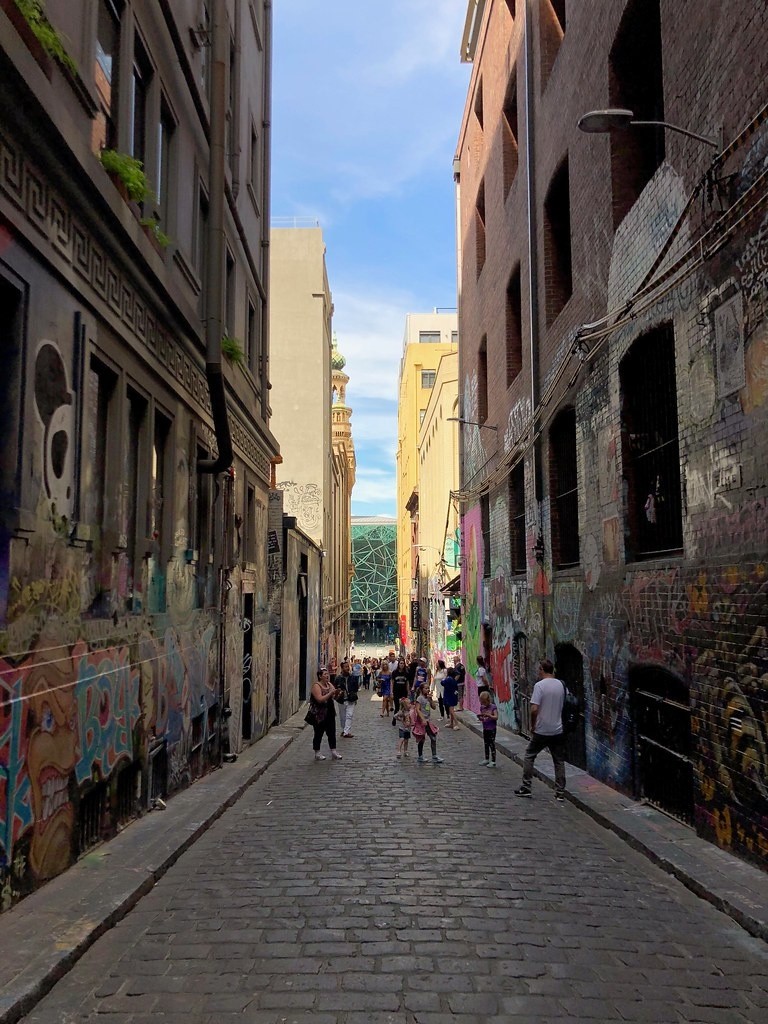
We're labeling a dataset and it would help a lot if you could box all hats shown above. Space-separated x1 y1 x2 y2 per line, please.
419 657 427 663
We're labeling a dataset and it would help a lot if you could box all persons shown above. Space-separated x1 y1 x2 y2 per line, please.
513 659 570 801
328 653 465 731
309 668 343 761
476 656 494 723
412 682 445 763
394 697 411 757
476 691 498 768
333 661 358 738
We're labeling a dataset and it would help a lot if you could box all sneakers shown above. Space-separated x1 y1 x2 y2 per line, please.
553 791 565 801
487 762 497 768
479 760 489 765
417 756 428 762
514 787 532 796
432 755 444 762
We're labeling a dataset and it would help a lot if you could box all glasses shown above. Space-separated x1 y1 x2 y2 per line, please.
342 661 348 663
320 667 327 671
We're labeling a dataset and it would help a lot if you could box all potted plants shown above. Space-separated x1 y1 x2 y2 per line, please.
0 0 77 81
222 337 247 371
100 147 170 261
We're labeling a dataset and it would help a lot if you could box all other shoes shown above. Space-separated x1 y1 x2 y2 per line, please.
445 724 453 728
380 714 384 717
390 707 394 711
385 713 389 716
392 719 396 726
340 732 354 737
437 717 444 721
333 753 342 759
447 716 450 721
403 752 410 756
315 753 325 760
397 751 401 758
453 726 460 731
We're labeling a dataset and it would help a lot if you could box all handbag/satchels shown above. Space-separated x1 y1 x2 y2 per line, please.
377 691 382 697
304 703 324 728
561 680 579 737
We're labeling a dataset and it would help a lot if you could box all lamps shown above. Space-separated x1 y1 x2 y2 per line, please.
577 110 718 150
447 417 498 431
